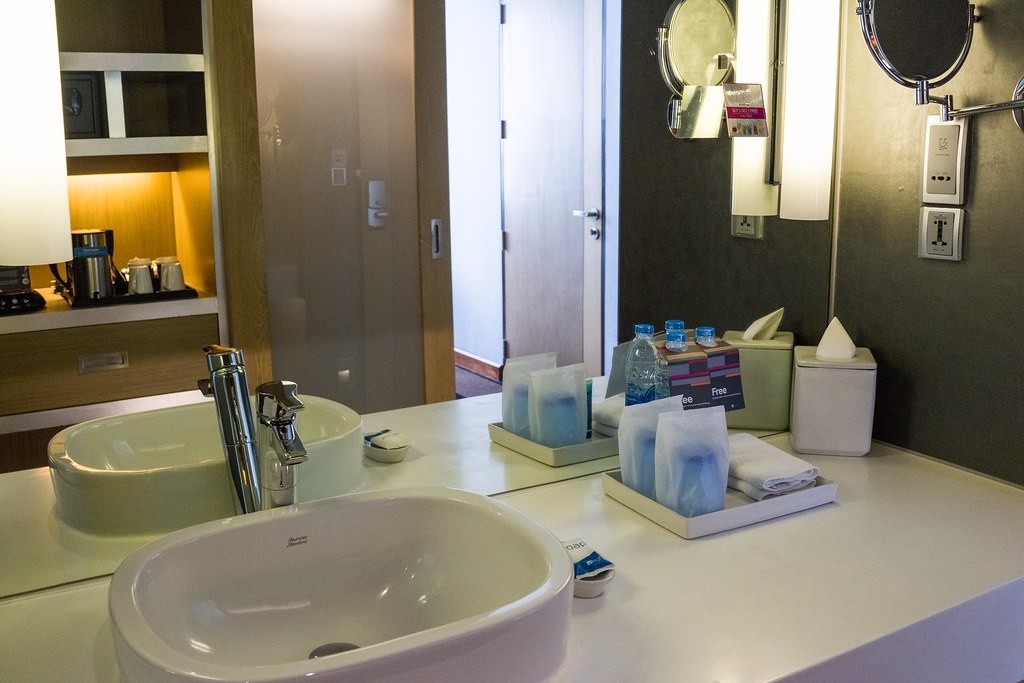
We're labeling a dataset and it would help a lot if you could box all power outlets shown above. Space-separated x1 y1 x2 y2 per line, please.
916 208 966 261
921 116 967 205
730 215 768 241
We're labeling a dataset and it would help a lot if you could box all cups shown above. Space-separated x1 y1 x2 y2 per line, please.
127 257 185 294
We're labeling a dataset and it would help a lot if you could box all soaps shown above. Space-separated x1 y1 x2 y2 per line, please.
558 536 617 581
361 424 410 453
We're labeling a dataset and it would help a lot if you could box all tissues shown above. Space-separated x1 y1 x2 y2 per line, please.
715 306 798 433
790 316 881 460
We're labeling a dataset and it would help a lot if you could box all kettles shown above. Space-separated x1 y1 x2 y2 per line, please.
49 228 115 299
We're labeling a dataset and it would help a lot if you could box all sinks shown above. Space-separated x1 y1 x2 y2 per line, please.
46 393 364 542
106 483 575 683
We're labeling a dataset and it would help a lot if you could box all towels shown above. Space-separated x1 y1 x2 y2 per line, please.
727 477 823 505
728 431 823 491
590 419 621 437
590 387 633 429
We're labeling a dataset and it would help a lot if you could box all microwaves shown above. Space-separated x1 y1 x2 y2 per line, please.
0 266 32 294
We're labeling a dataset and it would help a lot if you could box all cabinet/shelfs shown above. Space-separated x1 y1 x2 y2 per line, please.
1 0 232 474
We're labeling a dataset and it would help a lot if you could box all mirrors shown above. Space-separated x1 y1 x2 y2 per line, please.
658 0 736 138
857 0 1022 133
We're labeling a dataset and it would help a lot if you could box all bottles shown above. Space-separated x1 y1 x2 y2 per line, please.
654 326 717 400
624 320 684 407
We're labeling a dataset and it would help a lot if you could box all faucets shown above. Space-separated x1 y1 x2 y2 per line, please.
196 338 266 520
253 380 309 514
